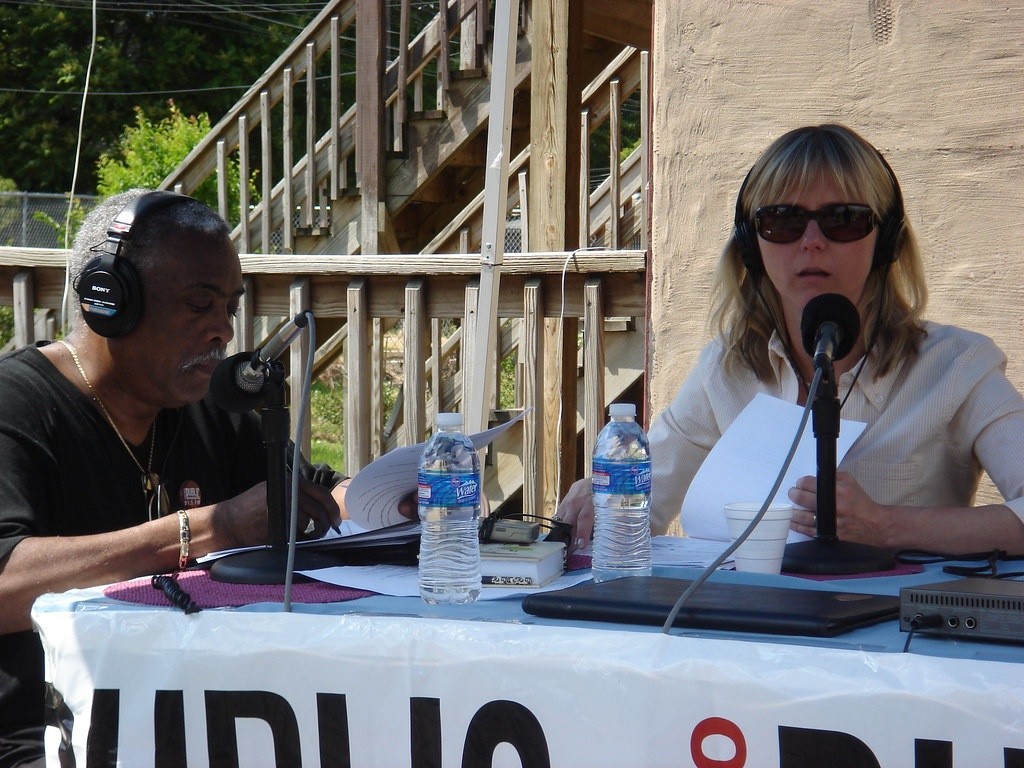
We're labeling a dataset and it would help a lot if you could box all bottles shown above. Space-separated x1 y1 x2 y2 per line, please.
418 412 483 608
591 403 653 582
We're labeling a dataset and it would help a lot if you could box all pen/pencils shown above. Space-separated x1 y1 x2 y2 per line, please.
286 462 343 536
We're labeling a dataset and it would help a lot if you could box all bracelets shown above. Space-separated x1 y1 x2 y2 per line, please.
178 509 190 570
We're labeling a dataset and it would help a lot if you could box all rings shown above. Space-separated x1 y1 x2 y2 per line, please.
813 514 816 523
552 515 563 521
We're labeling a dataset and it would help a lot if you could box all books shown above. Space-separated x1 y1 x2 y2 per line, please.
417 542 568 588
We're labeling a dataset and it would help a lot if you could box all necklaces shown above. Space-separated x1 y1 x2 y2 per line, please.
797 375 811 386
57 340 155 488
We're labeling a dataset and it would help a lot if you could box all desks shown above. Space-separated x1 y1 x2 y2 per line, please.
31 519 1024 768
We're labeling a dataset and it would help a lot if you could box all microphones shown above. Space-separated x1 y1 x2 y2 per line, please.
800 292 860 370
209 311 310 414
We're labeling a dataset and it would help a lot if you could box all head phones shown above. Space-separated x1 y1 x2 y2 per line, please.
75 190 209 338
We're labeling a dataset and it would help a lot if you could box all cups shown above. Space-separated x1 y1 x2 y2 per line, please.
724 500 793 576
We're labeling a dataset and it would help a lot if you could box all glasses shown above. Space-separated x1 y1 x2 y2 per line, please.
755 202 879 244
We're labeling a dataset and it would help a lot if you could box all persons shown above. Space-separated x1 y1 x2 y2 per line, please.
0 188 422 768
551 124 1023 557
